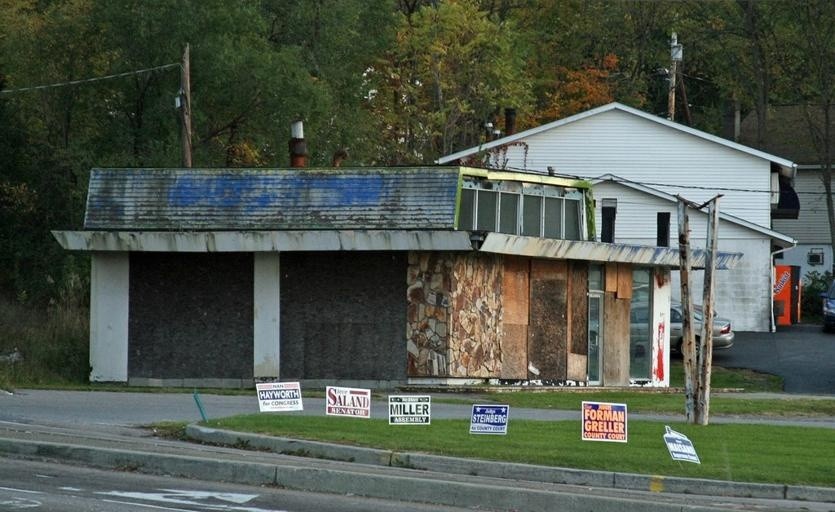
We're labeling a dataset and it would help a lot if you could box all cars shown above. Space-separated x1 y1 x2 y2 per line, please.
820 277 835 333
630 298 737 361
